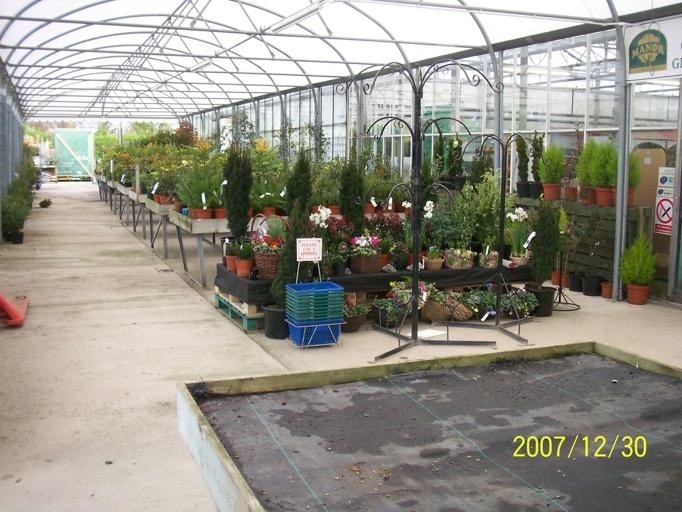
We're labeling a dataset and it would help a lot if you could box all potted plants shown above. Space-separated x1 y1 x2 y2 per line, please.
0 195 27 244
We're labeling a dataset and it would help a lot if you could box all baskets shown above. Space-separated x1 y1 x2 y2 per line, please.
253 215 289 279
285 281 345 345
